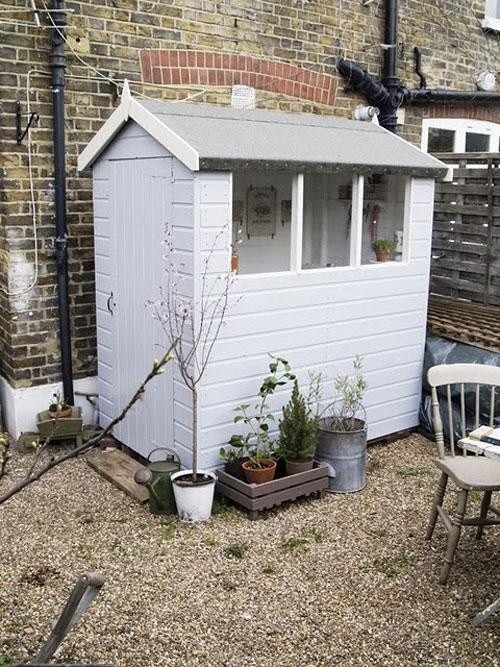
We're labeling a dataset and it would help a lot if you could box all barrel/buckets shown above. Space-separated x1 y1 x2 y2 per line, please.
314 399 368 493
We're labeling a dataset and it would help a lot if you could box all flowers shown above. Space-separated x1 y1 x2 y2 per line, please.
143 220 246 483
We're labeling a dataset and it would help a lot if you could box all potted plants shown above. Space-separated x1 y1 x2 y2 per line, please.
219 353 369 494
370 239 397 262
47 389 73 420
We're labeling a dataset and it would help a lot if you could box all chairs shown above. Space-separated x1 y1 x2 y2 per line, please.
425 363 500 586
